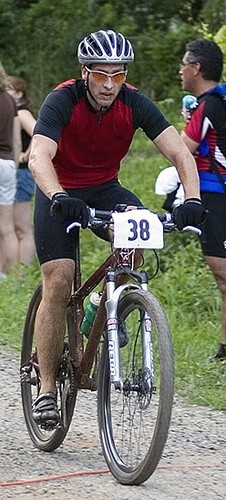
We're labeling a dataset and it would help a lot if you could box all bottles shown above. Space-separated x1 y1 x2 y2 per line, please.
80 291 104 338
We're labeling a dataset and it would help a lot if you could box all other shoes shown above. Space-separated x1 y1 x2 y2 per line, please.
208 345 226 364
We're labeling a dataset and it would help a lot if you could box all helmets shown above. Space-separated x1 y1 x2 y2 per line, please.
77 29 134 64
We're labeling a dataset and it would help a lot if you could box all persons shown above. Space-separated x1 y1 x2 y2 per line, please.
0 62 22 280
5 76 37 267
179 38 226 365
28 30 207 431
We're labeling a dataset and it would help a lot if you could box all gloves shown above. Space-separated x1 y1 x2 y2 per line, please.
174 198 204 230
50 192 88 229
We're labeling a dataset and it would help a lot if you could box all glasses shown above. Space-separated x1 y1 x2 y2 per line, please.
83 66 128 83
179 61 196 69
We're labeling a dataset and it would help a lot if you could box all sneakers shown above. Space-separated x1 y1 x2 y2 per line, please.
118 321 129 348
31 391 61 423
